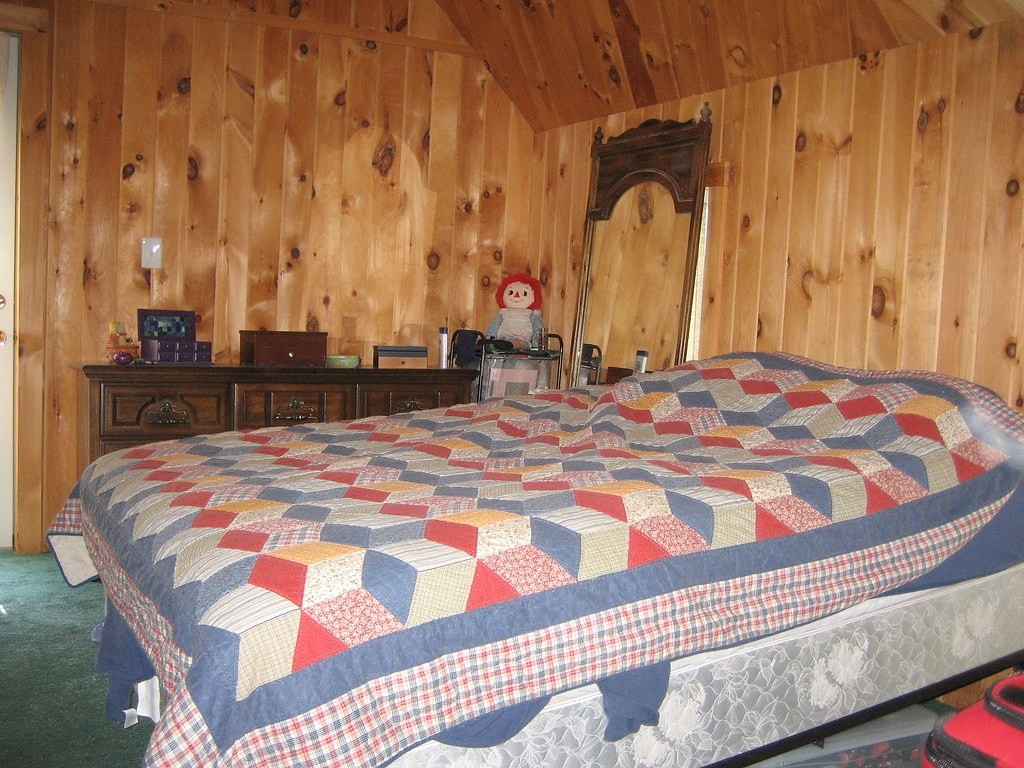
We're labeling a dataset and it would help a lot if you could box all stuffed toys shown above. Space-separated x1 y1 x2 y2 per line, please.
493 273 542 343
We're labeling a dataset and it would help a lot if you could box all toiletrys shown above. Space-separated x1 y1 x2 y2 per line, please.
438 327 448 369
633 349 649 373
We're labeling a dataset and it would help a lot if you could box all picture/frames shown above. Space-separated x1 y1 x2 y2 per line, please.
137 308 197 342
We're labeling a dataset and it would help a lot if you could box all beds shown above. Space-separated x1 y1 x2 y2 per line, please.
46 349 1024 768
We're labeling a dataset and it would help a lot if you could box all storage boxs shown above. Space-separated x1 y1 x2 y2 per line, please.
374 345 429 368
239 329 328 366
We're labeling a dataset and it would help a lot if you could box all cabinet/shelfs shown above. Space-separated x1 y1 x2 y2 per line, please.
450 331 565 402
82 363 478 463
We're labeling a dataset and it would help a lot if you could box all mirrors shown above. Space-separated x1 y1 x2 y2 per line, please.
568 102 715 402
